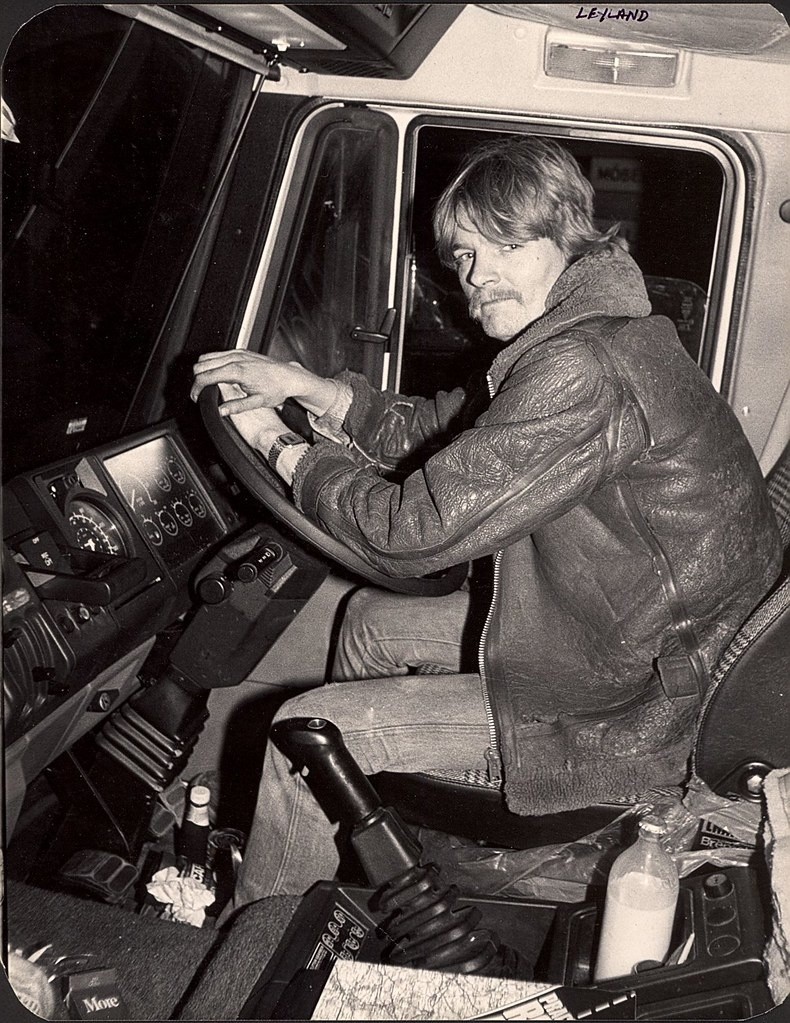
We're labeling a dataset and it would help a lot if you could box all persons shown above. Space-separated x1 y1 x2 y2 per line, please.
191 133 784 925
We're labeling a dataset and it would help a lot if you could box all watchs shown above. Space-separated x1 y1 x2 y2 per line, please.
269 432 310 471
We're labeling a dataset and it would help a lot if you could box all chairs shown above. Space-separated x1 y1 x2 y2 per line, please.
369 437 790 850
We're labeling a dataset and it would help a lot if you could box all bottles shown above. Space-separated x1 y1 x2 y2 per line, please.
595 814 679 981
179 786 211 866
